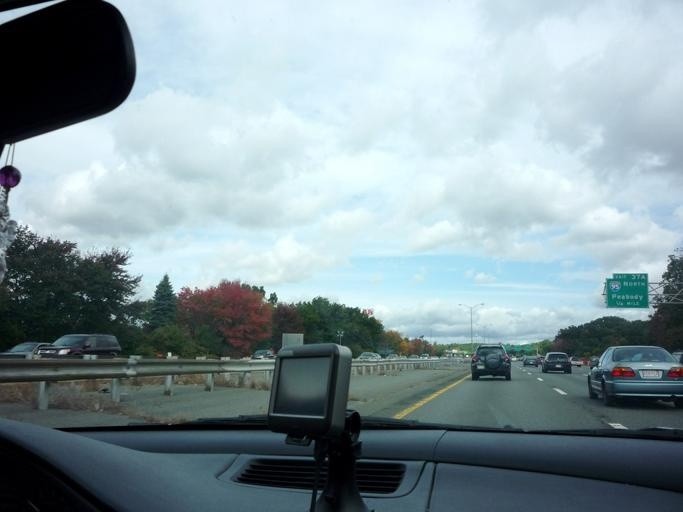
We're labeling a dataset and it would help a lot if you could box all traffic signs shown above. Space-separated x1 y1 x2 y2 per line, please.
609 279 622 292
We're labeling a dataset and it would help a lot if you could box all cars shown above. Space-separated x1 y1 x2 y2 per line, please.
541 352 572 374
0 333 124 360
251 349 274 360
510 356 516 361
587 346 683 407
523 355 539 368
470 343 511 380
355 350 470 361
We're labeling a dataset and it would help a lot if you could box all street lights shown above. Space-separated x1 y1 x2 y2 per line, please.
458 302 485 345
337 330 345 347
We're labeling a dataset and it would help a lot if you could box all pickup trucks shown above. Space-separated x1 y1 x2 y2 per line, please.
569 356 584 367
